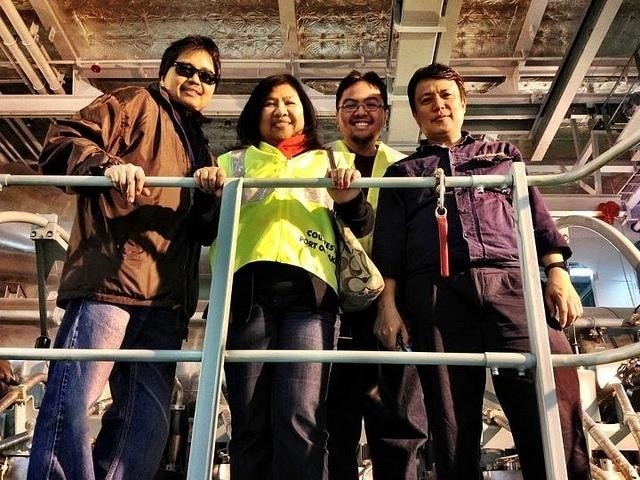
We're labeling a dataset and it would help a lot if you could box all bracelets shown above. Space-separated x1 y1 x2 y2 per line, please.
544 261 571 275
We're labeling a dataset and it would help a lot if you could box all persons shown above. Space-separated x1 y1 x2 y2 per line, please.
185 70 378 480
313 70 425 480
16 34 222 480
369 61 605 479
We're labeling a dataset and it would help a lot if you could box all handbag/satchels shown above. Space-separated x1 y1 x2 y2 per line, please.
334 214 386 313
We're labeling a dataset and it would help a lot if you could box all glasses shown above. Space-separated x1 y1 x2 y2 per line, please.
172 60 217 86
338 97 390 114
